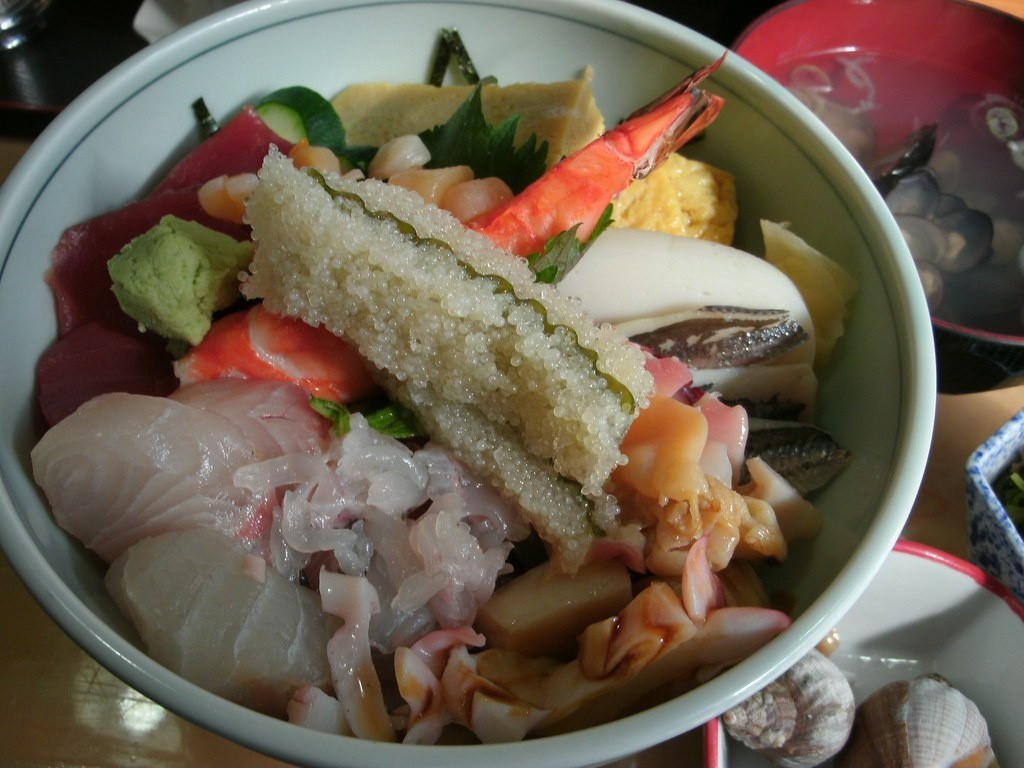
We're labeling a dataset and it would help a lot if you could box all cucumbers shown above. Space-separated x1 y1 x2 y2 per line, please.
250 86 347 156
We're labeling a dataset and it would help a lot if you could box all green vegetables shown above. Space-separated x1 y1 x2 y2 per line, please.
307 73 613 441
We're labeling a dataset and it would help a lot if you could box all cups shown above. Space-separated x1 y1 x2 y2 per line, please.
0 0 53 51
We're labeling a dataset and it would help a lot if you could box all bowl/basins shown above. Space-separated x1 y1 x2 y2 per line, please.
704 540 1024 768
730 0 1024 348
0 0 938 768
965 407 1024 602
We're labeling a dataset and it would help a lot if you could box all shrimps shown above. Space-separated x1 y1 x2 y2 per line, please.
171 46 733 408
